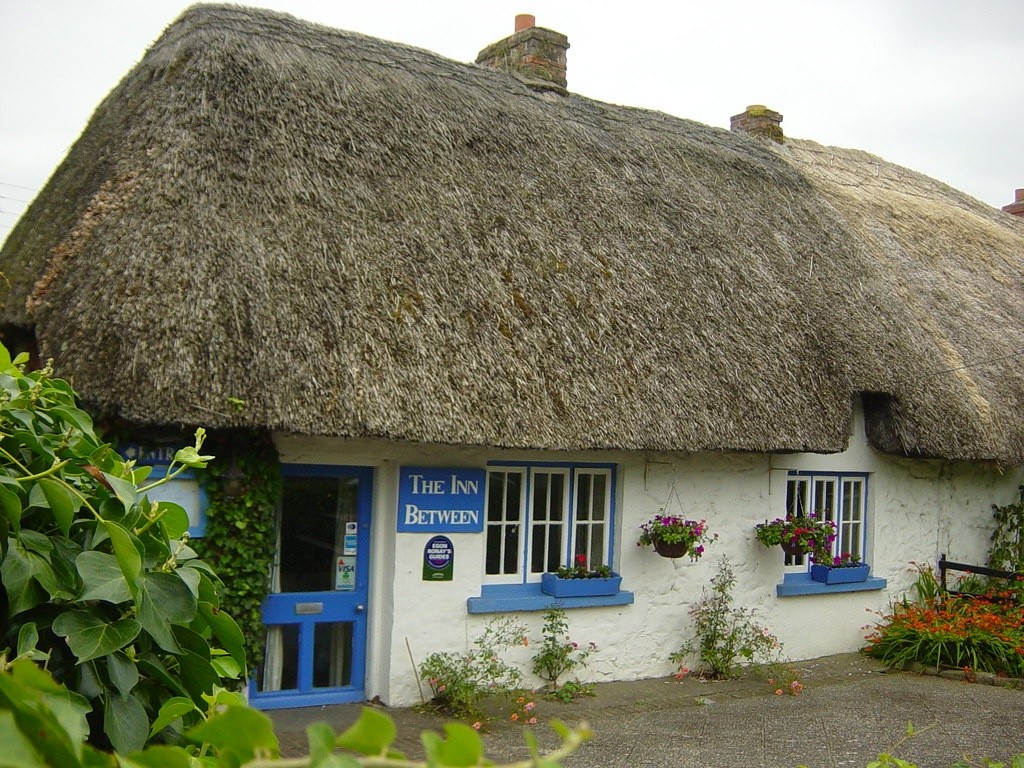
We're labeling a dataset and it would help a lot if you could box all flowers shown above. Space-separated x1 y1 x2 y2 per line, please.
831 552 862 567
558 554 613 579
755 508 836 563
636 513 720 562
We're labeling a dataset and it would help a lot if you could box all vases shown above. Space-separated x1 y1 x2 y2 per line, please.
811 563 871 585
542 572 623 597
781 541 805 557
653 536 687 558
